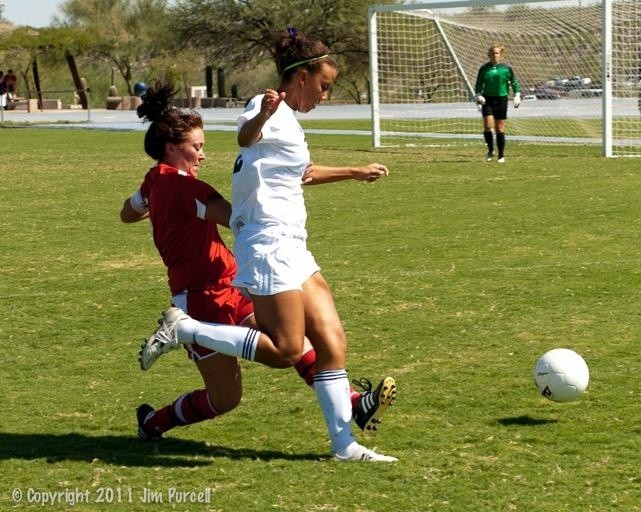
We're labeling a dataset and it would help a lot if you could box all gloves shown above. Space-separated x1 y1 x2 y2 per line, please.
476 95 486 105
513 93 523 108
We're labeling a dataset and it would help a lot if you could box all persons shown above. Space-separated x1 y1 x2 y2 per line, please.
475 45 521 163
0 69 17 123
120 28 405 464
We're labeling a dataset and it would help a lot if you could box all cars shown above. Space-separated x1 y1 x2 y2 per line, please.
523 76 603 101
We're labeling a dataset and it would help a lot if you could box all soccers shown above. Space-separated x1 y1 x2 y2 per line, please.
534 348 590 403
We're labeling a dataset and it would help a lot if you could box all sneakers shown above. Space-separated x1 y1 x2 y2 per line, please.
137 403 160 438
330 445 398 462
486 150 506 164
355 377 397 433
139 307 190 370
